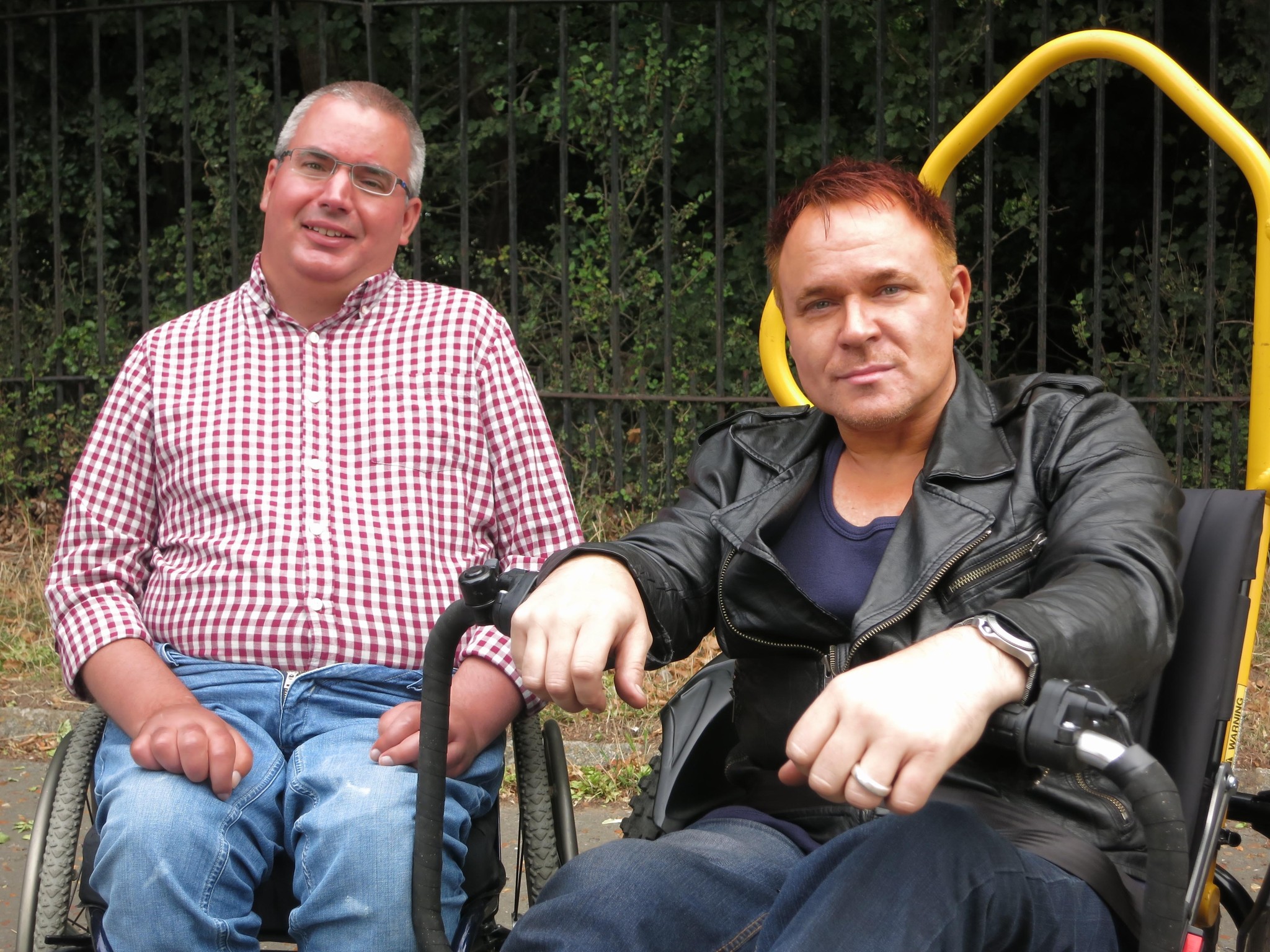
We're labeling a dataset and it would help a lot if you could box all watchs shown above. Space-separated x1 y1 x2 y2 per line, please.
948 611 1041 705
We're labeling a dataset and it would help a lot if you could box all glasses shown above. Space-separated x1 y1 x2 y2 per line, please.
278 148 412 200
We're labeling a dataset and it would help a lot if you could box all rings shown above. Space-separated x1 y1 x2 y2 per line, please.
850 763 893 797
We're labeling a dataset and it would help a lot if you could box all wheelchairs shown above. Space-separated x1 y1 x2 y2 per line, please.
14 584 580 952
412 476 1270 952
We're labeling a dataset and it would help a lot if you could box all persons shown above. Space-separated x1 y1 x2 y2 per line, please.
501 153 1185 952
44 81 587 952
